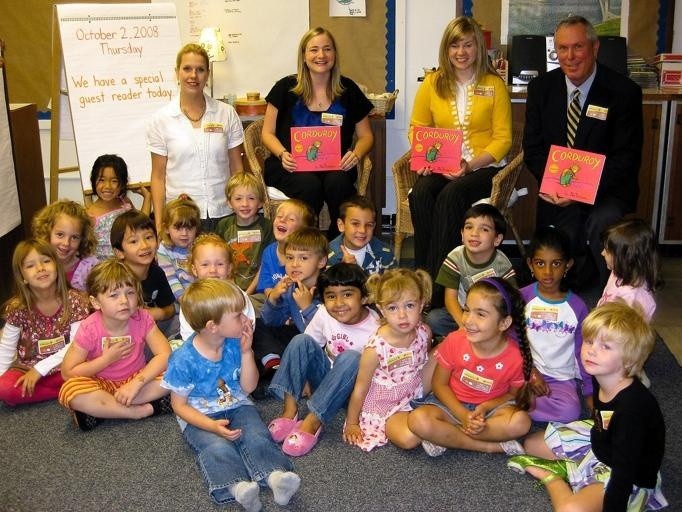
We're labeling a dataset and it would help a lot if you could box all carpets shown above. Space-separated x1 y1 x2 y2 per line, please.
0 324 682 512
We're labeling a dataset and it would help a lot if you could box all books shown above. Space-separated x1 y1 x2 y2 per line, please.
410 126 462 174
540 144 607 205
290 125 342 171
625 55 658 90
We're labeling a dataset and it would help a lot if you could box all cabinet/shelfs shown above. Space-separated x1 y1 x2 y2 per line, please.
506 90 681 251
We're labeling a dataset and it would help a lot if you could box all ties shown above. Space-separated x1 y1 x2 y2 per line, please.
567 89 582 149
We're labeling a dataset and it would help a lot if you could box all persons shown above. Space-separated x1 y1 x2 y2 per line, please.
2 150 433 451
408 16 512 307
261 26 375 241
407 203 666 456
158 275 301 512
522 15 644 296
150 43 244 239
506 296 669 511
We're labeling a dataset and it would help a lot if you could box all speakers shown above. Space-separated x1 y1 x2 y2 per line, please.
508 35 546 85
597 36 627 76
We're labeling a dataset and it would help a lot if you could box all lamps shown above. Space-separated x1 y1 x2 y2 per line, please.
198 27 228 99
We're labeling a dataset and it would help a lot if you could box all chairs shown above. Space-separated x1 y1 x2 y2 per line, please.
243 119 373 235
392 121 530 269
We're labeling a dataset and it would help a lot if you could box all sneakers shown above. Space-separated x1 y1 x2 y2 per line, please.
149 394 173 418
282 420 324 457
505 455 567 486
422 440 447 458
74 411 105 430
268 412 299 443
500 440 525 456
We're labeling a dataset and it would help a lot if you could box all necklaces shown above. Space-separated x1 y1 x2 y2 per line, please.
316 100 324 107
184 108 206 121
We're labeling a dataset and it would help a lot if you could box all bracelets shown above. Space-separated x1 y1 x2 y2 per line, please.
279 148 288 161
